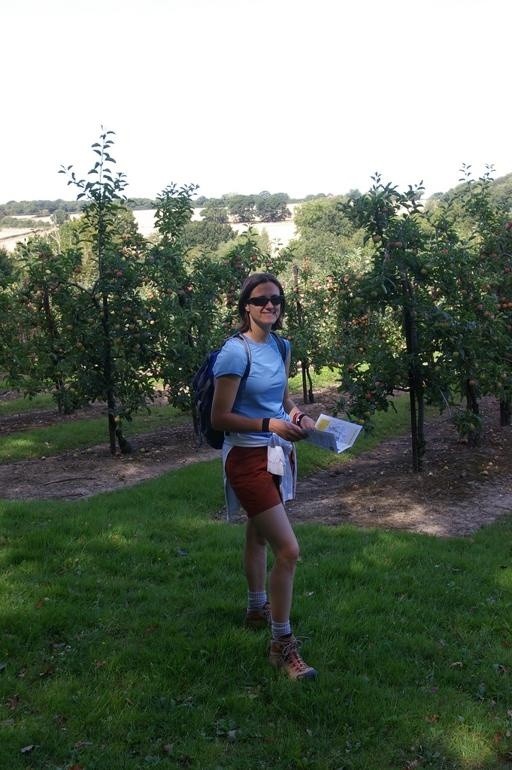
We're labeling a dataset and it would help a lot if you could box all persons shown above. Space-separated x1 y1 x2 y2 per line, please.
209 274 318 679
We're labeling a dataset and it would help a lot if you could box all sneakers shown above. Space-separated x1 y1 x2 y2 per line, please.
269 631 317 682
243 602 271 627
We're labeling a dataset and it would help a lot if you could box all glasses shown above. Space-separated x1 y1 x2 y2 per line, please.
246 296 282 306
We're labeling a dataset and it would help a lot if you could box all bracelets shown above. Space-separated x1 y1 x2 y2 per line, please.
261 417 270 433
293 411 300 424
296 413 311 428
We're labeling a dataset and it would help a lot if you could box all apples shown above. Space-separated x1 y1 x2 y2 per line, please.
21 221 512 432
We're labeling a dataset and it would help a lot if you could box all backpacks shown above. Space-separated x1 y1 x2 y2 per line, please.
192 331 286 450
